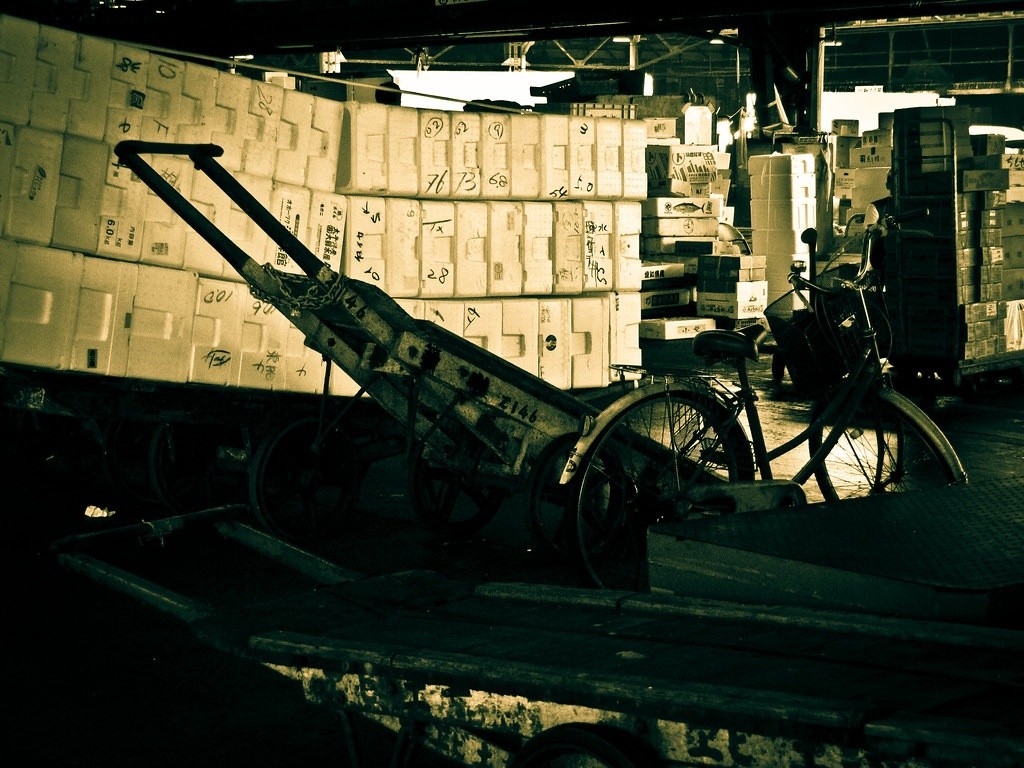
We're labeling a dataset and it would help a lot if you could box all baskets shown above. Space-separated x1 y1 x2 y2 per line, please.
763 264 889 396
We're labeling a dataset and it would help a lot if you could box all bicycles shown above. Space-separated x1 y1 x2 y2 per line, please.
556 207 971 592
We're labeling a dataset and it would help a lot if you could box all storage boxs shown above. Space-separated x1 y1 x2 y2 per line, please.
0 13 1024 403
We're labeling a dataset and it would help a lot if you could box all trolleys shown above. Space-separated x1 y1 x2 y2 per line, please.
112 140 735 565
